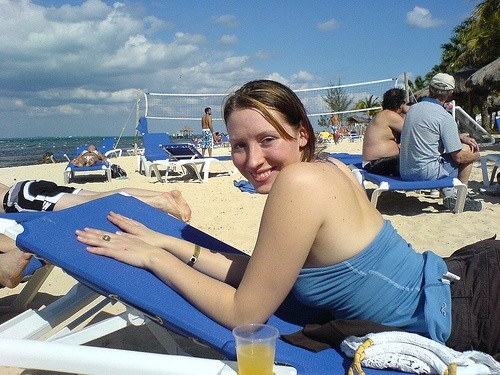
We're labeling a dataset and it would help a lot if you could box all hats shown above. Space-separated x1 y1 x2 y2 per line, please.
429 72 456 90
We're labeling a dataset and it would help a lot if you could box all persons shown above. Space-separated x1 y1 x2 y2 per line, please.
75 79 500 358
0 179 191 224
329 112 341 145
192 137 202 143
214 132 221 142
362 88 410 179
0 233 33 289
72 145 108 168
201 108 219 157
400 73 482 211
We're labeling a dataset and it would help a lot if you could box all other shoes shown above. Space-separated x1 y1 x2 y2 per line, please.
444 197 482 211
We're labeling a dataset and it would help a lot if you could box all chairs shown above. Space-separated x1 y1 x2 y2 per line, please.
159 143 234 184
482 154 500 194
0 211 61 282
138 133 181 178
64 146 110 184
315 129 363 143
97 137 122 158
326 152 466 214
0 191 419 375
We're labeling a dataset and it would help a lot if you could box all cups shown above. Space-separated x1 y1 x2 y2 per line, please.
232 323 279 375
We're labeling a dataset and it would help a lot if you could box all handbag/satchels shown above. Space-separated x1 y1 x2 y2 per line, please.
111 164 127 178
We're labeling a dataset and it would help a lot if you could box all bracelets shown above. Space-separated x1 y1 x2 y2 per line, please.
187 245 200 267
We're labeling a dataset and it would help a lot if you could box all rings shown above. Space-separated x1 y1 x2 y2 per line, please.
103 235 110 241
